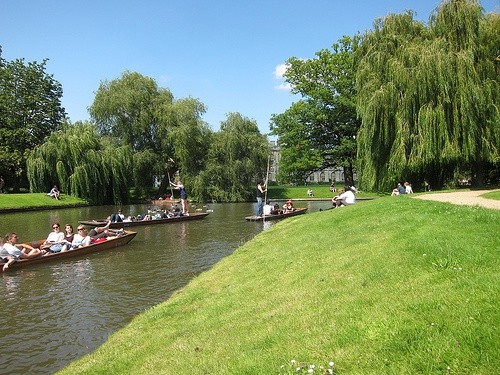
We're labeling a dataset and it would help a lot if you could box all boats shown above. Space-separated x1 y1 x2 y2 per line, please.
0 229 137 272
245 208 307 221
78 211 209 228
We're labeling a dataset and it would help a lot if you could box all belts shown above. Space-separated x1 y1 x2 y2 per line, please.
49 250 60 253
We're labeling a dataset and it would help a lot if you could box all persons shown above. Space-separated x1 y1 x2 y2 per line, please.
136 205 184 221
424 178 431 192
282 201 295 214
490 175 498 187
62 224 91 252
107 208 132 222
329 182 336 193
88 220 111 240
170 181 189 214
0 238 15 271
262 201 272 215
273 204 283 215
320 186 357 211
307 188 314 197
40 223 75 253
5 233 46 259
391 183 405 196
404 182 413 193
256 180 267 216
50 186 60 201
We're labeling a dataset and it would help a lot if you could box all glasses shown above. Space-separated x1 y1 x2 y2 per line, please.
53 227 58 229
78 229 83 231
